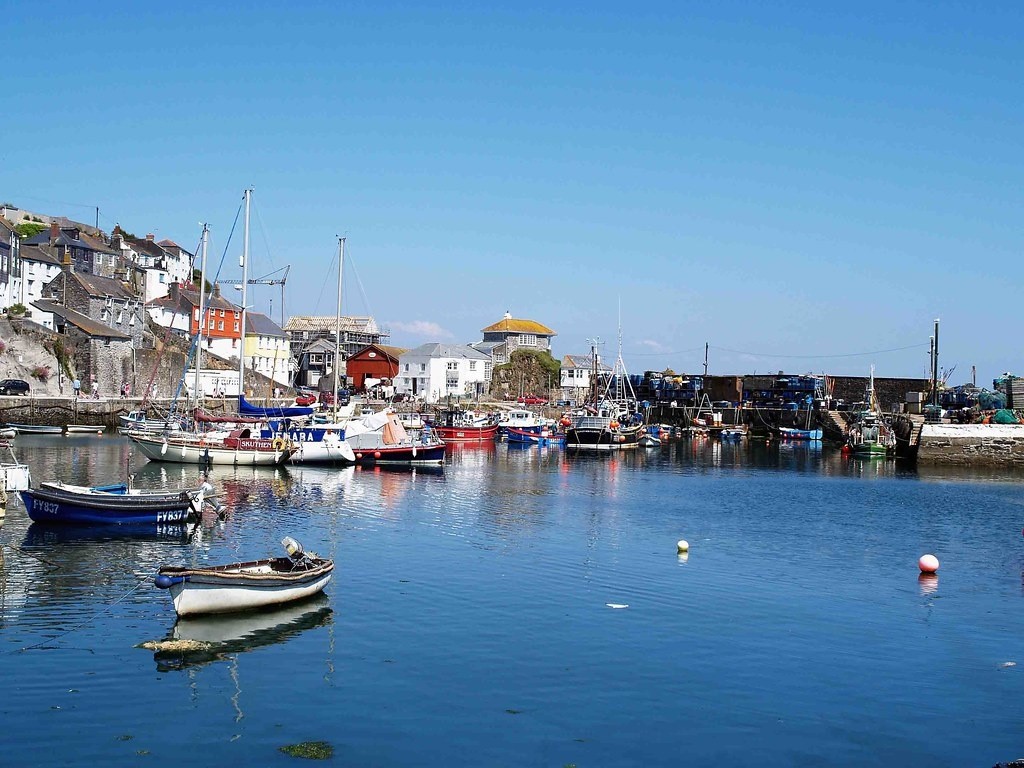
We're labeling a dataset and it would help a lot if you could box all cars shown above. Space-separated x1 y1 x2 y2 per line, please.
297 386 312 394
319 390 331 403
555 399 567 406
326 396 340 411
295 393 315 406
384 393 410 403
517 395 548 404
338 390 350 406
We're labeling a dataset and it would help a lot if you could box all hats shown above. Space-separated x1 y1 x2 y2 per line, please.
126 382 129 384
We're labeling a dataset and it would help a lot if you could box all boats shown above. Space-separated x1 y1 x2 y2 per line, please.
778 425 823 440
152 536 335 618
842 363 897 456
6 422 63 434
153 591 334 674
18 480 205 527
66 424 107 432
0 427 18 439
20 520 202 548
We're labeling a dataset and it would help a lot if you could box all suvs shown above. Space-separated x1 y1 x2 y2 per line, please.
0 379 30 396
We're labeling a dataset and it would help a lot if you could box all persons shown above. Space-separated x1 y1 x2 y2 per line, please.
91 379 99 400
488 408 500 425
73 378 80 397
196 477 212 491
120 381 130 399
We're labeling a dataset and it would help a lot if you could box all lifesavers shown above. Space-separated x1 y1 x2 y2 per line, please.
272 439 285 452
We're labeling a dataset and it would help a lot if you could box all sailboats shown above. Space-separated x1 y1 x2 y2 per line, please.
114 183 749 476
